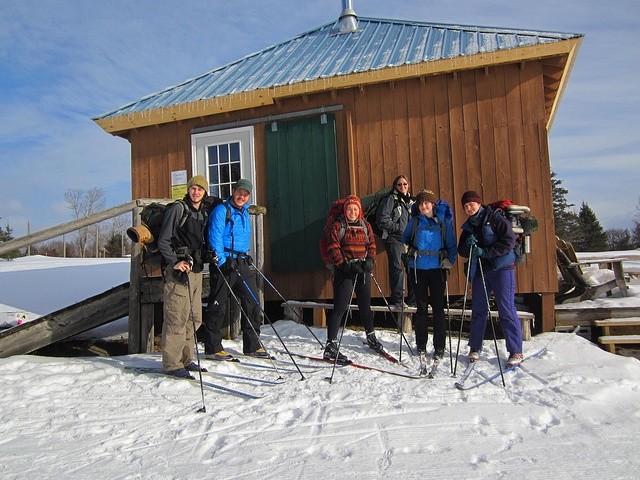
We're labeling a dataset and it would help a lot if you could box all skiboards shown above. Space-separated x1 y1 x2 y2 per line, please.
125 366 290 399
195 354 347 373
419 354 441 379
281 337 422 379
455 348 548 390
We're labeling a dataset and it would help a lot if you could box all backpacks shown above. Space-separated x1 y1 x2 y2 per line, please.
488 200 538 265
139 199 208 266
320 199 369 270
200 196 246 263
363 193 414 239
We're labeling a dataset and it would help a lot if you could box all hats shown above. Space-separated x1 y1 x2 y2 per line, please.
233 179 252 194
343 195 364 226
461 191 482 206
187 176 208 192
416 189 435 203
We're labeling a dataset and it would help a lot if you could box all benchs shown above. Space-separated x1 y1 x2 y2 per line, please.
555 234 632 300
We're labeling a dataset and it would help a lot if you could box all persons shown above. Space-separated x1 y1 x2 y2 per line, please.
403 188 457 360
158 174 209 381
376 174 419 310
458 191 524 368
324 195 384 364
204 179 275 361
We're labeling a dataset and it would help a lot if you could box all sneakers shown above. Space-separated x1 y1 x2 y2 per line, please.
417 347 426 354
507 353 523 365
185 363 199 370
169 369 190 377
206 351 233 360
434 349 444 359
367 331 383 350
244 348 268 356
468 350 480 360
394 302 408 310
323 339 347 362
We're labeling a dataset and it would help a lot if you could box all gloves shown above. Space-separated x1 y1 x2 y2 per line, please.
473 247 484 258
172 253 194 283
221 257 239 270
247 256 253 266
343 263 363 273
363 258 374 272
465 235 478 245
440 258 452 270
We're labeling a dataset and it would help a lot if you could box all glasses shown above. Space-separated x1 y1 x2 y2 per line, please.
398 184 407 186
